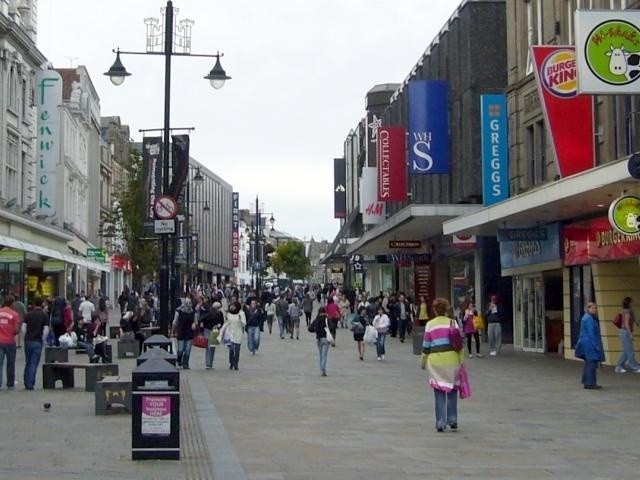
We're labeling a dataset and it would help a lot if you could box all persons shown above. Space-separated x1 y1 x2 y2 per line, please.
43 291 160 360
462 301 483 359
308 308 335 376
484 294 504 356
613 296 640 373
2 296 20 386
171 284 262 370
351 307 370 360
12 295 27 348
419 296 465 433
373 306 390 360
22 298 50 391
573 302 606 387
261 282 357 347
357 289 427 343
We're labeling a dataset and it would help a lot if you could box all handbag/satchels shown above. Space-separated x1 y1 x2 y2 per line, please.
450 319 462 351
613 313 622 329
324 317 335 345
472 313 485 329
193 331 208 348
458 366 471 399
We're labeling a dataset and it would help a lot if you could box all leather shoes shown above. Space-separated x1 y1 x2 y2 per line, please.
584 384 601 389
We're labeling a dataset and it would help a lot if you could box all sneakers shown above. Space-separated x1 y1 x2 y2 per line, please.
632 368 640 373
381 354 384 359
614 368 627 373
377 357 381 361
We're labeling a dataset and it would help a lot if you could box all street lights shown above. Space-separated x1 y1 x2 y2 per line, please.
102 0 230 331
175 198 216 292
239 206 276 298
148 161 204 307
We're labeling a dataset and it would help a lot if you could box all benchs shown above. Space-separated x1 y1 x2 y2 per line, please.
41 346 132 416
